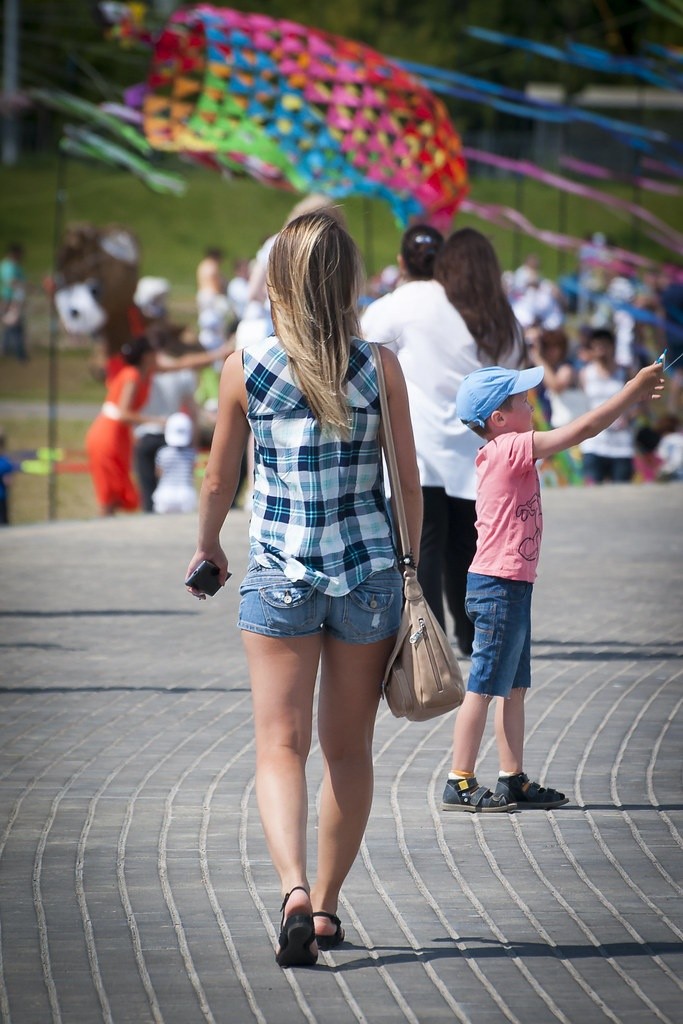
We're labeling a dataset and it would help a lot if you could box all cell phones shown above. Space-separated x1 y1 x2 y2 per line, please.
184 560 232 597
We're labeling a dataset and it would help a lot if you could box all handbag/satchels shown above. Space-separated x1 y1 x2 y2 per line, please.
380 570 465 722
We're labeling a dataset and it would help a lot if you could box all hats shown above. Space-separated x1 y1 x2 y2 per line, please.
456 365 547 429
164 412 193 448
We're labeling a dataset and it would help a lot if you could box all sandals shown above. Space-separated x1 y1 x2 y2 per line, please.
443 779 517 812
312 911 344 950
496 773 570 808
276 887 318 966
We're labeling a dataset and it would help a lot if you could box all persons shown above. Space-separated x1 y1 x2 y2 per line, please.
442 361 666 813
0 220 683 528
184 210 423 967
355 226 528 657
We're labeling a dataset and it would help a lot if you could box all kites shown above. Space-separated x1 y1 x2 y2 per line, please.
0 2 683 344
